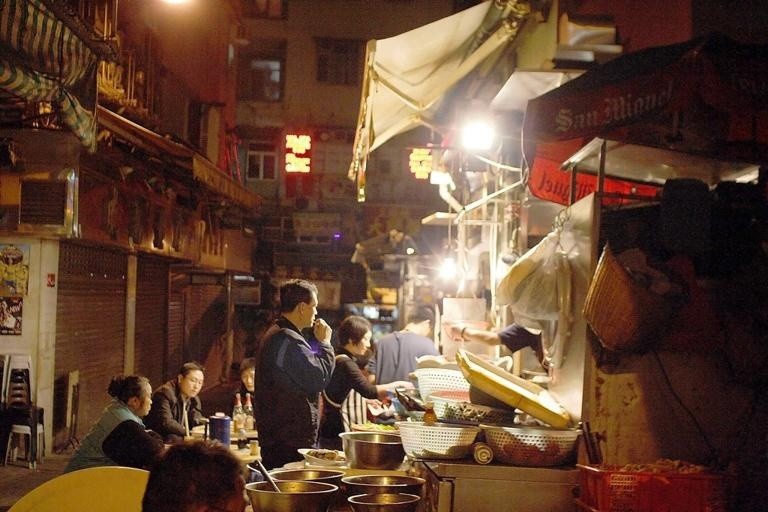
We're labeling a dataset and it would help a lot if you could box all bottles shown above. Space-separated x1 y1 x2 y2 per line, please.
233 391 255 435
250 440 259 456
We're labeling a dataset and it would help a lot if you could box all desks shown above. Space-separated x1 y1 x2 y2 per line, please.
58 369 80 455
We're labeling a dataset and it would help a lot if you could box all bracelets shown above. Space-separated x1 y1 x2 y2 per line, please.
461 326 468 338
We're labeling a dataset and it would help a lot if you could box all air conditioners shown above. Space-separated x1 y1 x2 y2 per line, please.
293 195 321 213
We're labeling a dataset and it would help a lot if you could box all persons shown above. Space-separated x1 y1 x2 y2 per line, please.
255 280 336 471
314 316 415 450
364 304 440 386
143 439 246 511
450 322 549 373
62 374 164 475
142 363 210 444
227 358 255 420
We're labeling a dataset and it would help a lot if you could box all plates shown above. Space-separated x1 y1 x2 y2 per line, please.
296 447 345 466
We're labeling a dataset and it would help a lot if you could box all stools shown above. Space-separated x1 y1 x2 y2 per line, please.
0 351 46 470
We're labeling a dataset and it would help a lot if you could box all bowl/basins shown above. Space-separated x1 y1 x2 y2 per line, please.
338 430 407 472
243 467 429 512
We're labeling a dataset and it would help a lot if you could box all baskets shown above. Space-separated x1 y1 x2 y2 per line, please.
582 241 669 352
573 462 732 512
478 425 584 466
397 420 480 460
415 367 515 426
